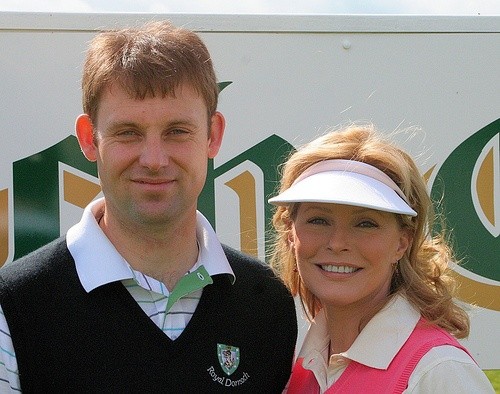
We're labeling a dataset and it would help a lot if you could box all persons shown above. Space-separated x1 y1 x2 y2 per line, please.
263 123 496 394
0 20 296 394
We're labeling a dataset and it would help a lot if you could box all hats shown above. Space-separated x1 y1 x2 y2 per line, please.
267 158 419 218
